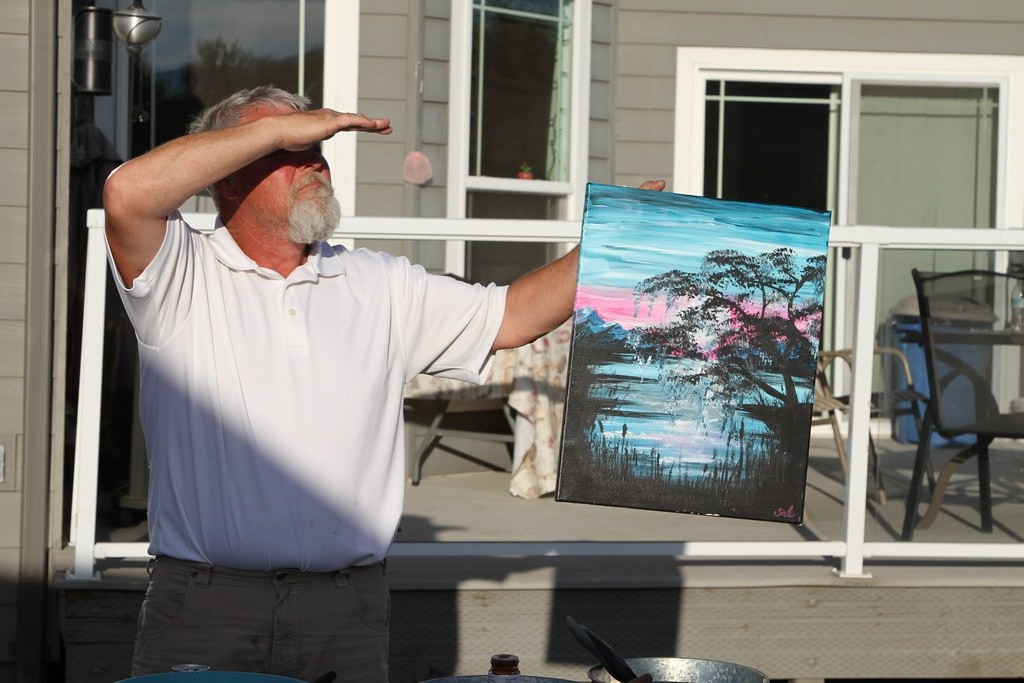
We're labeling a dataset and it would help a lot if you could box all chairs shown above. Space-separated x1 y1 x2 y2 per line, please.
901 267 1024 543
813 349 935 505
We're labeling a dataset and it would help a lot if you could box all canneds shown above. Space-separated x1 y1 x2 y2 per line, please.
172 663 210 672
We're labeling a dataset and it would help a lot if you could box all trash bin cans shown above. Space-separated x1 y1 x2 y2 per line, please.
881 293 999 446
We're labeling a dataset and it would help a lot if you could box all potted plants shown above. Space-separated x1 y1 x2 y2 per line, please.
517 161 535 179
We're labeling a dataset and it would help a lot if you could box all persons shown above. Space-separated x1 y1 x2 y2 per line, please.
104 83 666 683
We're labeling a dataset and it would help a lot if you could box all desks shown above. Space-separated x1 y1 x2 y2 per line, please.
403 317 574 499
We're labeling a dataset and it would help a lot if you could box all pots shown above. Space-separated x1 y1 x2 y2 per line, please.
587 657 770 683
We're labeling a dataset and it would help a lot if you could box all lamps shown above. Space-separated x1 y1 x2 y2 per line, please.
71 0 163 94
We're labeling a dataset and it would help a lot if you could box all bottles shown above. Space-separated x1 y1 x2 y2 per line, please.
1010 278 1024 334
